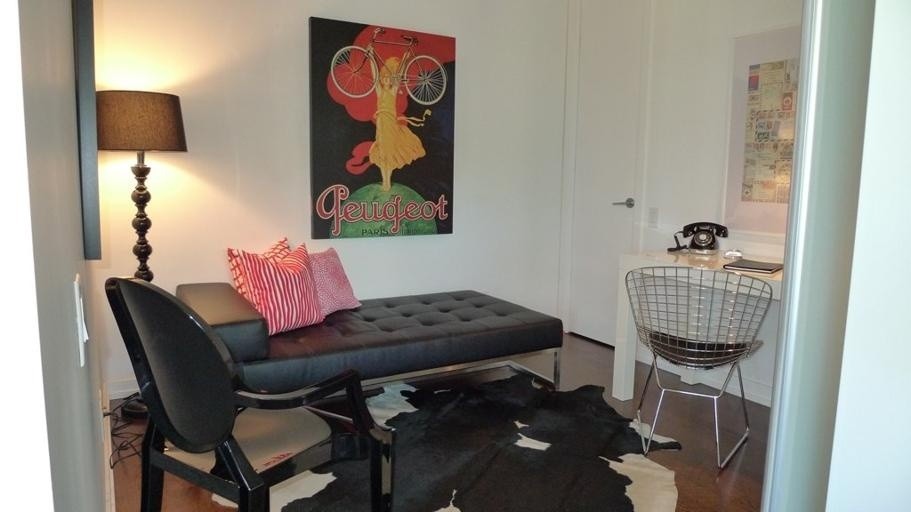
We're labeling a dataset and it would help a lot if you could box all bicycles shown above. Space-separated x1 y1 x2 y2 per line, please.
332 28 447 103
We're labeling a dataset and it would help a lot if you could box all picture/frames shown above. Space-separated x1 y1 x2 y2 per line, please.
717 19 802 246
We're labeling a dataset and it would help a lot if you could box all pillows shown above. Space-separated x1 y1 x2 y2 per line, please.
227 236 362 337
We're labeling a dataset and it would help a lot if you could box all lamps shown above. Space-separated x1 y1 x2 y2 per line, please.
96 90 187 418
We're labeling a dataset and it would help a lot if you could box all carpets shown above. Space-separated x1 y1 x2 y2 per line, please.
164 360 679 511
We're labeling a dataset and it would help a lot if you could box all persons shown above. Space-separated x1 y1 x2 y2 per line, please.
366 44 432 192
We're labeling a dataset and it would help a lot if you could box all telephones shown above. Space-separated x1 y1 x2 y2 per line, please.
667 222 728 255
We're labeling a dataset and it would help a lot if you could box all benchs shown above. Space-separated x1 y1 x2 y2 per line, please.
177 282 564 394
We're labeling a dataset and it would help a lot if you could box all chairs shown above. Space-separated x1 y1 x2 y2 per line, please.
105 276 397 512
624 266 773 469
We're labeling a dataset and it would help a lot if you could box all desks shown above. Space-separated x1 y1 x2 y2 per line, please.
611 250 784 402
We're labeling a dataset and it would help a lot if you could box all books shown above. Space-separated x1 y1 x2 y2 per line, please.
724 258 783 274
